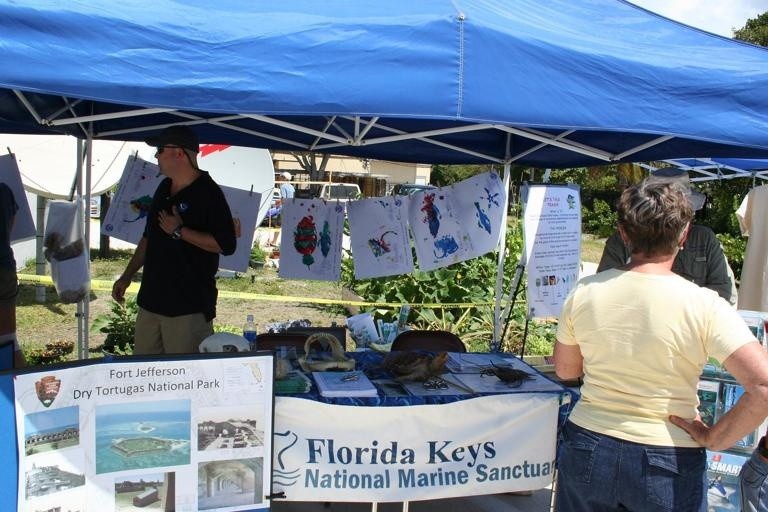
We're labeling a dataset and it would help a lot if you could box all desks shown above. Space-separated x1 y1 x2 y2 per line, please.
270 352 572 512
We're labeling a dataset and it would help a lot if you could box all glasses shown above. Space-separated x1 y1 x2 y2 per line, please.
156 146 184 154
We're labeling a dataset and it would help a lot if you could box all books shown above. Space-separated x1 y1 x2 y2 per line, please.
310 369 378 400
274 368 311 394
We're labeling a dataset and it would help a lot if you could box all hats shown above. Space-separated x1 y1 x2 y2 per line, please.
650 167 706 211
144 125 199 153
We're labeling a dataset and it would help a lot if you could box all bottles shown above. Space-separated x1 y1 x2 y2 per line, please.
243 315 256 353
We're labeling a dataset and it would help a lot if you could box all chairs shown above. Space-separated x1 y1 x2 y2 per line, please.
286 328 347 352
257 334 313 351
197 332 250 354
392 332 467 351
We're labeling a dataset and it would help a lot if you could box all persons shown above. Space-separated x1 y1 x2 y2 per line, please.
596 168 737 313
0 183 29 369
548 180 767 510
737 435 767 511
109 124 238 358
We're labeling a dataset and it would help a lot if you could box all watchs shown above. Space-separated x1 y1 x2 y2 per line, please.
170 223 187 242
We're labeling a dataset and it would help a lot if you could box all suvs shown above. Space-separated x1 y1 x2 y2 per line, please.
319 183 362 219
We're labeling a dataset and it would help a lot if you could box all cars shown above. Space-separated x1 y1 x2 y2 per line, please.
389 184 437 197
